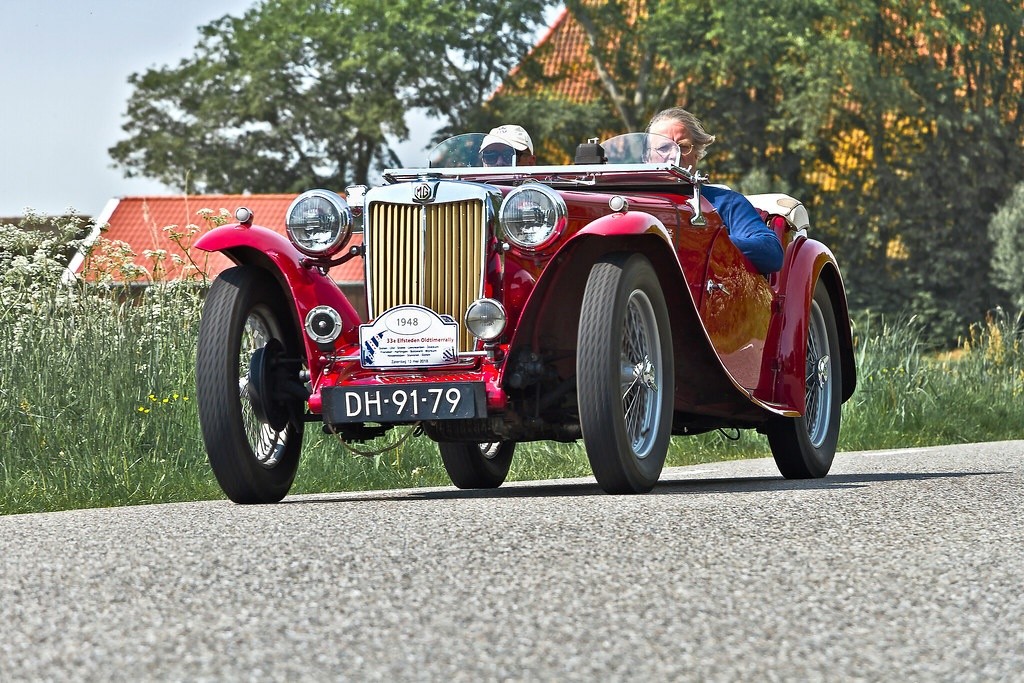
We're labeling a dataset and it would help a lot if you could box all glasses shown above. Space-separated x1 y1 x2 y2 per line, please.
648 141 698 156
483 149 531 164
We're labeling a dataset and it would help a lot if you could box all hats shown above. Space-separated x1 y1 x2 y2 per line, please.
479 125 534 155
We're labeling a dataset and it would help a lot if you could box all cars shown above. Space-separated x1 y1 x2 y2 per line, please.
197 133 858 504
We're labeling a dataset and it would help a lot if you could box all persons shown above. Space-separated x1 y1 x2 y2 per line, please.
478 124 537 167
641 107 785 275
